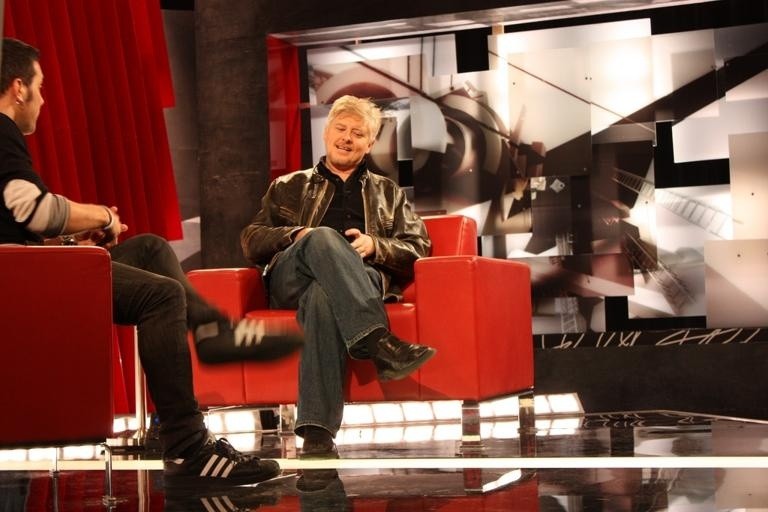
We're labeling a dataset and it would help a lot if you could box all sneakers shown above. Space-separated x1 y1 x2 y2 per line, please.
166 436 278 488
166 491 277 511
195 317 296 364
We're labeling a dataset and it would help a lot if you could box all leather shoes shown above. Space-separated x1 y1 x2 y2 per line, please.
299 430 339 459
372 328 436 382
296 470 337 494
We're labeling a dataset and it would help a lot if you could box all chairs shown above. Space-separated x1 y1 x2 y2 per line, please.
184 216 534 444
1 245 112 503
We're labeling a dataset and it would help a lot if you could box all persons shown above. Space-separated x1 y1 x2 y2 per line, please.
0 34 307 489
156 481 282 512
290 463 351 511
239 93 440 457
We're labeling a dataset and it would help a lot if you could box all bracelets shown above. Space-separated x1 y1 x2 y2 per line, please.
103 206 115 232
